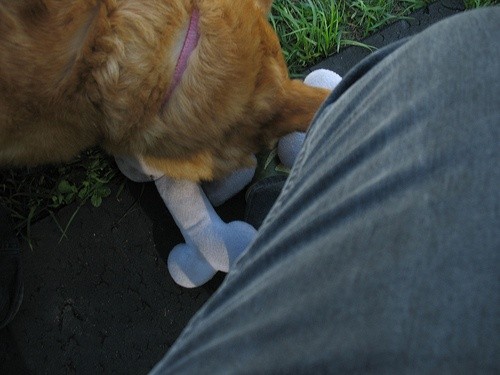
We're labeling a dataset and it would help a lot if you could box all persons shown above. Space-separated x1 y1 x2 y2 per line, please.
148 6 500 375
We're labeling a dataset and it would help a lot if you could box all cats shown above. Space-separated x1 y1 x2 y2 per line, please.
1 0 335 186
